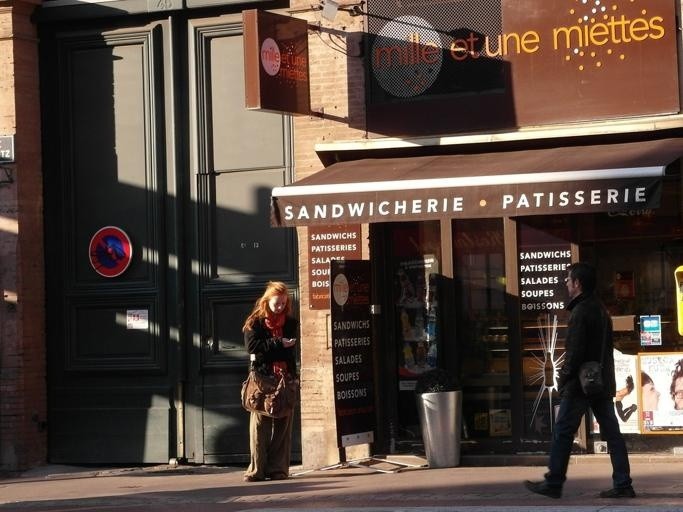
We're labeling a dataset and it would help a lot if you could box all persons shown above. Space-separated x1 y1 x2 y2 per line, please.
614 376 638 422
243 282 299 482
641 360 683 430
524 263 635 499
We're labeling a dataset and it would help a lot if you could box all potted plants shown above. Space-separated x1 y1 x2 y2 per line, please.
416 384 463 468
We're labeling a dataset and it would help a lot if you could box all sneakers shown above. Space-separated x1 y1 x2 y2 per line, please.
600 485 636 498
524 479 562 498
244 472 288 482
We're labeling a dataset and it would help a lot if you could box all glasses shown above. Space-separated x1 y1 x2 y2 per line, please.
564 278 572 282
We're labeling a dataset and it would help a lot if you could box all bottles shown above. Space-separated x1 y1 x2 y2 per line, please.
399 308 413 340
544 351 555 388
426 342 437 368
401 342 415 368
426 306 438 341
414 342 426 368
414 307 426 340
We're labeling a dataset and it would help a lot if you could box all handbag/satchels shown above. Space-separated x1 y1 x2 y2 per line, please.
240 369 299 418
579 362 604 396
